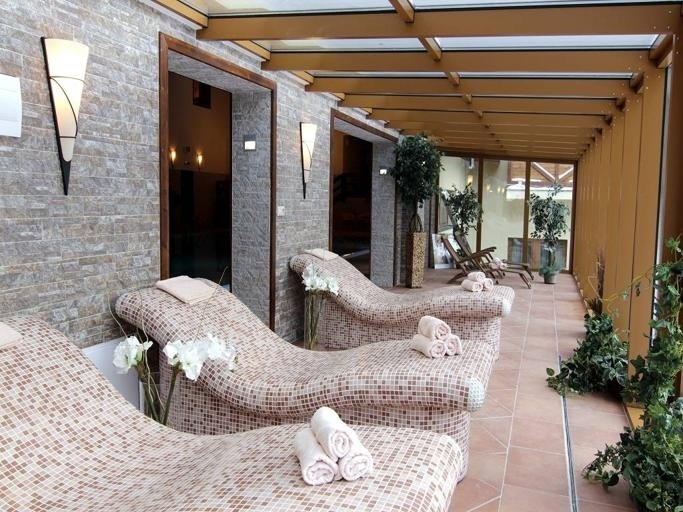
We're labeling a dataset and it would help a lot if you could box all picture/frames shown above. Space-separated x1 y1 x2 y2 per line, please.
191 79 213 113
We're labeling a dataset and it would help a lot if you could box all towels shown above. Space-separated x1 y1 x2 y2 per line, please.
411 316 462 359
294 406 373 486
304 246 338 261
488 257 507 270
461 271 494 292
156 275 216 304
0 321 25 350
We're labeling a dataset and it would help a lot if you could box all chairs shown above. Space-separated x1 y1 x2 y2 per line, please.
440 234 532 289
114 277 496 484
290 251 515 361
454 229 534 280
0 314 463 512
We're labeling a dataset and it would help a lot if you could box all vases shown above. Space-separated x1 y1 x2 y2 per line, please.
139 372 187 431
303 290 327 352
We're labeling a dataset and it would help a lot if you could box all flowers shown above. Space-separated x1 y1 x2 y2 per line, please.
293 236 340 349
99 266 237 425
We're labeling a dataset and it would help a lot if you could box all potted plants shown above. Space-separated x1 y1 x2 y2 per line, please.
582 232 683 512
443 182 484 269
526 182 571 284
544 298 630 398
388 131 445 289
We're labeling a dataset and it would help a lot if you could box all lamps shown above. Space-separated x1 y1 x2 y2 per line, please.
300 121 318 199
41 36 91 195
195 151 203 169
168 145 177 166
